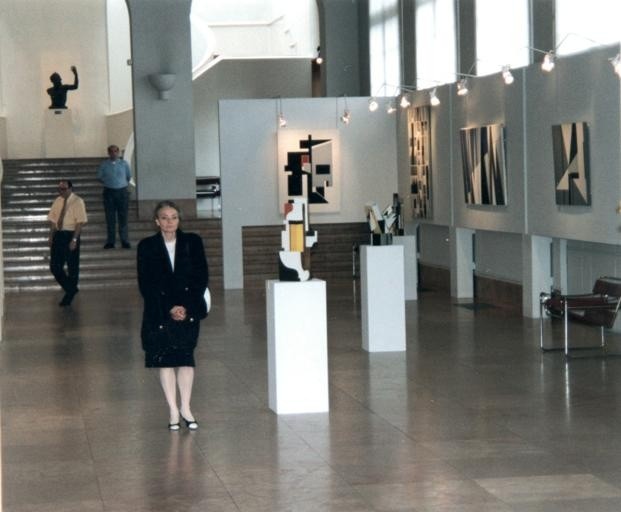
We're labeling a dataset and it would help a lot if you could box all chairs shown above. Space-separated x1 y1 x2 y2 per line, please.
196 176 221 211
540 276 621 359
352 231 395 276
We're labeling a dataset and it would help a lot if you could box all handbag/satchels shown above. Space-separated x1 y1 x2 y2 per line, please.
197 287 212 318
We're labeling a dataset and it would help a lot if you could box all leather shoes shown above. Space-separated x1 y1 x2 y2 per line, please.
57 287 79 305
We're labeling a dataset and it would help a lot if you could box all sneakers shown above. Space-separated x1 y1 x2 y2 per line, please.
122 242 130 248
104 243 114 249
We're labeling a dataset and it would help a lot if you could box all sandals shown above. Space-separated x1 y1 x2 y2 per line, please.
168 422 181 431
180 411 199 430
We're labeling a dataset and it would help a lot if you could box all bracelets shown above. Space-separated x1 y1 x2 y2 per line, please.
72 238 76 241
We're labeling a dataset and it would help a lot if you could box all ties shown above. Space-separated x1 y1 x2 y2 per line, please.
58 198 67 230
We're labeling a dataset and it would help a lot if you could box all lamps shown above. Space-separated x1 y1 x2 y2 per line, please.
335 49 556 125
277 95 291 128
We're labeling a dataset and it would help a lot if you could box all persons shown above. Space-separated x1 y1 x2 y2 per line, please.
47 181 88 305
136 200 212 430
96 145 132 249
47 66 79 108
